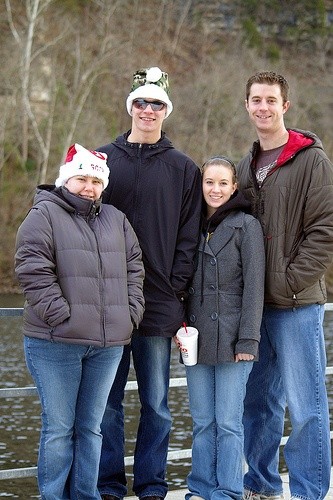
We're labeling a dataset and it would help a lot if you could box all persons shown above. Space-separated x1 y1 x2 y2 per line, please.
172 155 267 500
207 72 333 500
91 67 202 500
14 142 146 500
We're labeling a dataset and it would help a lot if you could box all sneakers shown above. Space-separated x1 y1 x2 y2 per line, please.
242 489 282 500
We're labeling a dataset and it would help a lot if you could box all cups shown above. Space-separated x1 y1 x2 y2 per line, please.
176 327 199 366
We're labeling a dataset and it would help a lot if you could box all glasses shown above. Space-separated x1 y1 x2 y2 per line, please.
133 98 165 111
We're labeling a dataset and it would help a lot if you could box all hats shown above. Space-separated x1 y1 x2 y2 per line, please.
55 143 110 189
126 67 173 120
